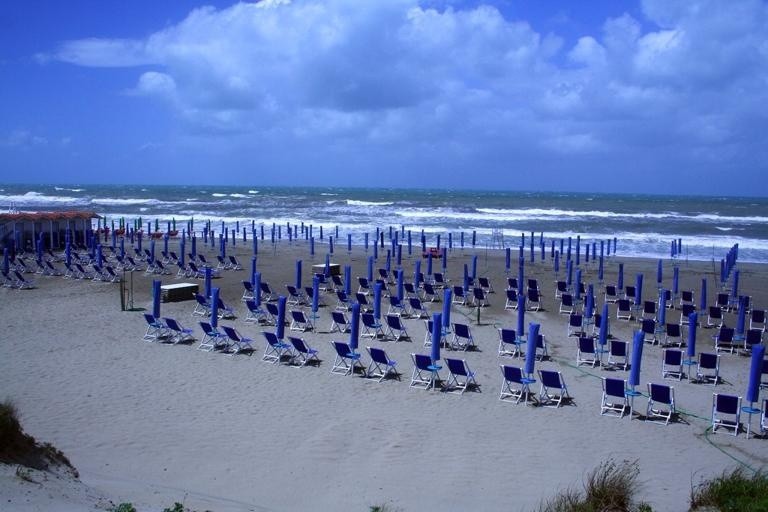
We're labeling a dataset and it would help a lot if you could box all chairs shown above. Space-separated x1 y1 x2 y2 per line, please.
330 311 352 334
574 337 600 369
499 364 529 403
450 322 475 352
744 327 764 354
661 348 686 381
600 376 630 419
134 292 243 357
365 345 399 383
260 332 292 364
289 310 314 332
244 281 254 296
536 369 570 409
497 328 522 358
335 291 352 312
607 340 630 372
759 398 768 433
640 318 657 345
313 266 345 291
525 332 548 360
1 245 243 293
265 303 288 325
696 352 721 385
423 320 442 348
568 313 585 338
286 285 305 306
355 266 543 320
382 313 408 341
760 360 768 390
712 392 742 437
553 279 768 331
286 335 321 367
645 381 677 425
664 323 684 348
712 327 735 355
360 313 384 339
442 358 479 392
244 300 267 325
408 352 442 390
303 286 326 307
330 340 365 378
592 313 611 337
260 282 278 302
